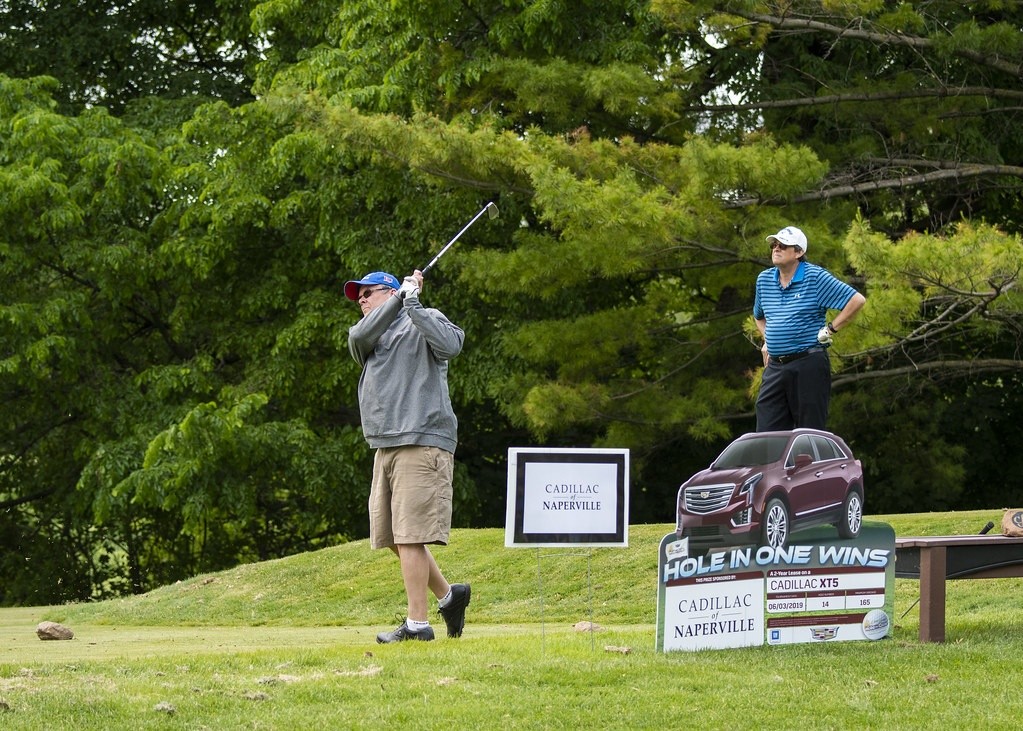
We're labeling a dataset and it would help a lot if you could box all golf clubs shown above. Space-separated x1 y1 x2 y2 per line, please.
900 521 995 619
399 201 499 300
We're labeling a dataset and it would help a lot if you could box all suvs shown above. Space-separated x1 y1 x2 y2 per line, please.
676 428 864 550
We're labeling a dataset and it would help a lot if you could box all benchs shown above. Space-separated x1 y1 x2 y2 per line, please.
894 535 1022 641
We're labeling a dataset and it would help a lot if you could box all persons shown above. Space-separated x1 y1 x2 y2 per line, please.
344 269 471 644
752 225 866 431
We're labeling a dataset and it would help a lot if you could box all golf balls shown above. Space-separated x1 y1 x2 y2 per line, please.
861 608 890 641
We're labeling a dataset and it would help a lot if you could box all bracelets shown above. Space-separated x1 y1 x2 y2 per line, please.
828 323 838 333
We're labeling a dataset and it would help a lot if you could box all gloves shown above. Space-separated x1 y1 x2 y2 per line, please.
817 326 834 344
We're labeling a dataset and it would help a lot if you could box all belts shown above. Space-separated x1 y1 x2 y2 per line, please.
771 347 826 363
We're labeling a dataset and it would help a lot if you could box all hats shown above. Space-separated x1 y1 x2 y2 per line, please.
344 271 400 302
766 226 808 252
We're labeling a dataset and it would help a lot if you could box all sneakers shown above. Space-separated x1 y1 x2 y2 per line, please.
376 613 435 644
437 583 471 639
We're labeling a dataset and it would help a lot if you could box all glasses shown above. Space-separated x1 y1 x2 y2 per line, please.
769 242 795 250
357 287 392 300
